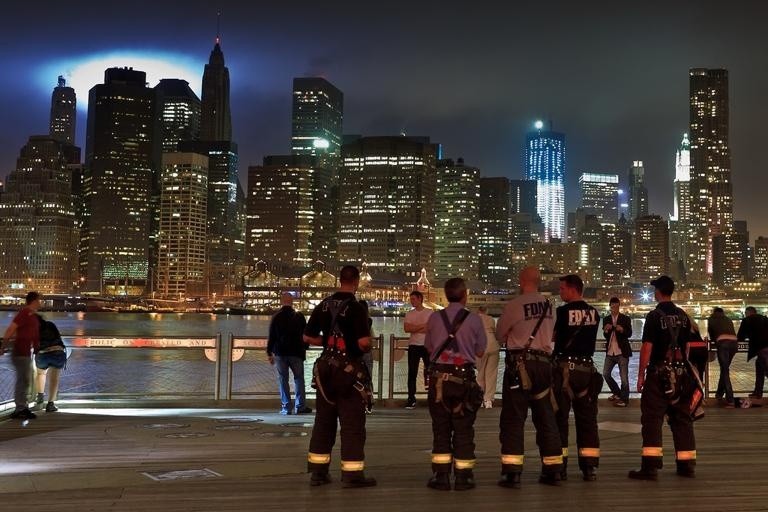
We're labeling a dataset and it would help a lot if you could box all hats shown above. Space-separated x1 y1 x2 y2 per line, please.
650 276 674 293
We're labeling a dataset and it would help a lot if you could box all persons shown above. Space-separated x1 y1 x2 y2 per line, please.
265 291 313 416
0 291 43 419
301 263 379 488
600 275 768 482
493 265 565 490
422 276 487 490
474 305 500 410
551 272 601 483
33 312 67 413
403 289 435 411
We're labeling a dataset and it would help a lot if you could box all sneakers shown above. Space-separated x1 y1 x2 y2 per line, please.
609 394 627 407
715 394 734 410
426 472 474 490
682 470 696 478
539 472 566 487
279 409 291 414
480 400 492 408
628 470 658 480
582 466 597 482
406 402 416 409
342 477 375 488
748 394 761 398
296 407 312 414
9 402 58 420
310 473 331 485
498 475 521 489
